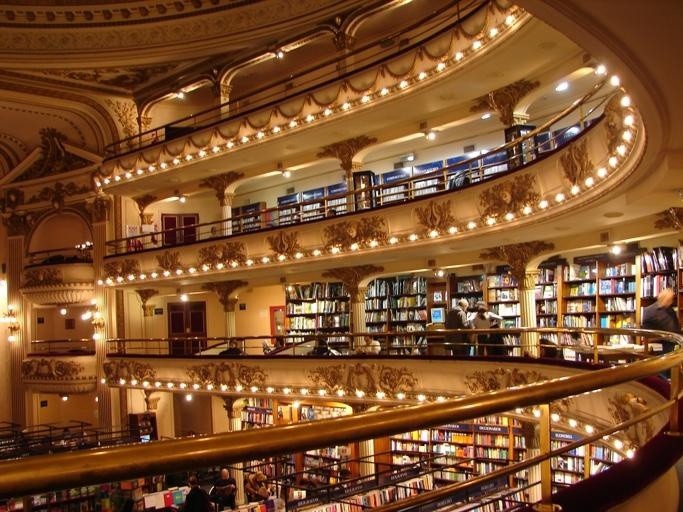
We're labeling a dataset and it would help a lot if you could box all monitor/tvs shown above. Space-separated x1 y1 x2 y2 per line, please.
430 308 445 323
140 434 151 443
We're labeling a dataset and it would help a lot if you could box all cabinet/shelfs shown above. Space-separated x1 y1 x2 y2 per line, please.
232 243 679 512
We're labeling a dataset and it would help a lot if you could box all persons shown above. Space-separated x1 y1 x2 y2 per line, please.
488 274 521 356
180 475 209 511
644 288 681 356
264 334 284 355
539 339 556 356
209 226 221 239
447 300 503 356
210 468 236 511
219 340 247 356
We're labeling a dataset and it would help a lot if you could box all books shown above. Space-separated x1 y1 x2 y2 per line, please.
310 474 431 512
390 429 529 500
0 472 196 512
285 282 355 355
551 441 623 495
231 195 346 237
562 264 596 357
641 248 683 322
241 446 350 511
378 160 507 206
241 407 344 430
453 279 481 356
365 278 427 356
453 489 525 512
599 261 637 362
533 269 558 345
